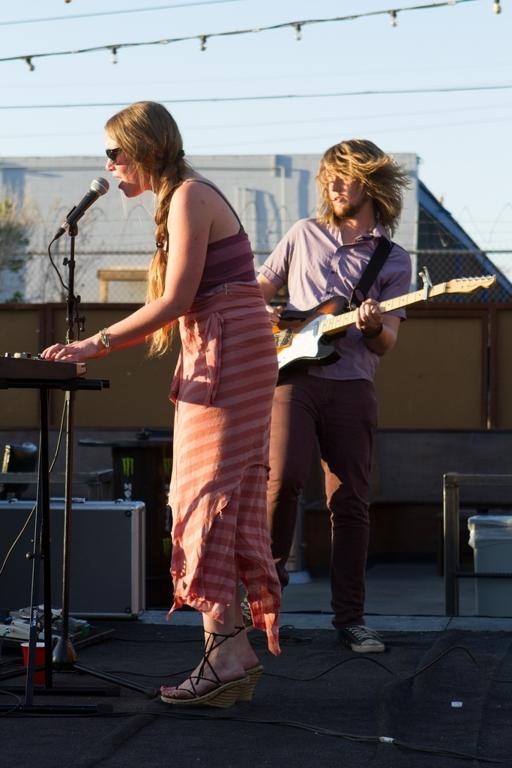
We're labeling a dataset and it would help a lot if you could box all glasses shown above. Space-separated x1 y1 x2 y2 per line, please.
105 145 124 162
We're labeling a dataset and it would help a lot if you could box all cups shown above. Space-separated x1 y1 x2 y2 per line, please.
20 642 49 688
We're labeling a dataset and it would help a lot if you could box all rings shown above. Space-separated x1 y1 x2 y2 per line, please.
364 320 369 323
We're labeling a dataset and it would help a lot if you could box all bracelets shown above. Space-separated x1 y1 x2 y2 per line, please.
362 325 382 339
98 328 111 357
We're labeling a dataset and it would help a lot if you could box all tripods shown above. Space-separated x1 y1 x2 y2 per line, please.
0 233 159 698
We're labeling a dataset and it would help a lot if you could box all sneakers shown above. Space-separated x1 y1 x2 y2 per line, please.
240 598 257 634
334 620 387 654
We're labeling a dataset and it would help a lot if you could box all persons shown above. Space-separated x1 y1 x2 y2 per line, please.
243 138 412 654
39 100 283 708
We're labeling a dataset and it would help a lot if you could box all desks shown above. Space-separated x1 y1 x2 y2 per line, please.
77 437 173 608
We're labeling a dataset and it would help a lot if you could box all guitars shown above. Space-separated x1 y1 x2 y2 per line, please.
272 264 496 386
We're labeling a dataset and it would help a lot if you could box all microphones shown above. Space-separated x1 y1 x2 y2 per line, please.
55 176 110 242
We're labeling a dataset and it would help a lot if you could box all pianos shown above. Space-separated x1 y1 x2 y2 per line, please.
1 352 88 381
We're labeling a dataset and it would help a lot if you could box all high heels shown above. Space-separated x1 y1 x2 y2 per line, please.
158 662 271 710
